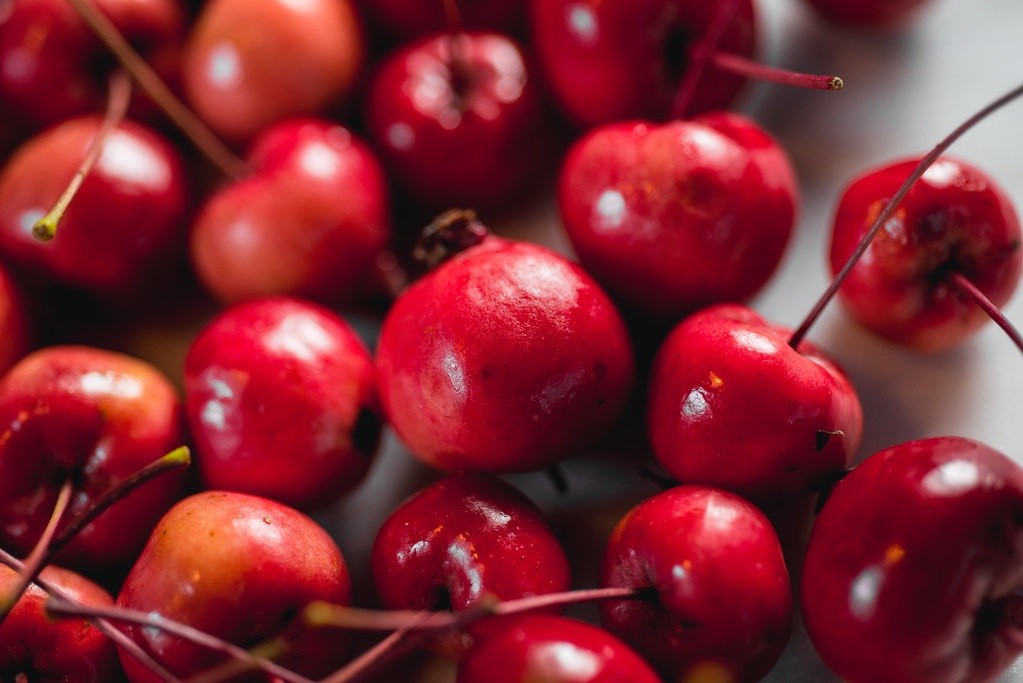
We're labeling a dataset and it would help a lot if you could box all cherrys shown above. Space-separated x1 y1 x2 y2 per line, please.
0 0 1023 683
